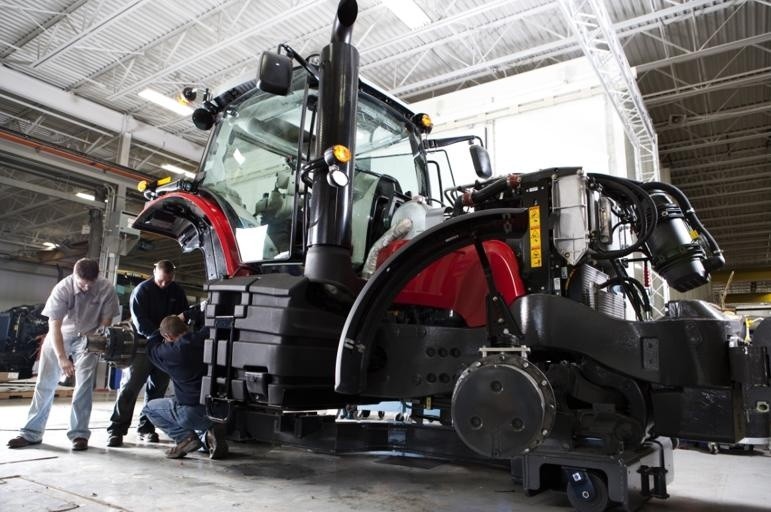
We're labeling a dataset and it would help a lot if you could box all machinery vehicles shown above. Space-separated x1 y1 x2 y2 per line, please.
78 2 771 510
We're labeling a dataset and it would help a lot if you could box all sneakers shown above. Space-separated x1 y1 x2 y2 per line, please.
190 441 199 445
104 432 123 447
6 435 44 449
138 429 160 442
164 434 203 461
207 425 230 459
71 438 89 451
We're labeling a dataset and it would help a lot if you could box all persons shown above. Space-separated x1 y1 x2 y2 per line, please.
143 308 228 459
7 257 120 450
107 259 189 447
